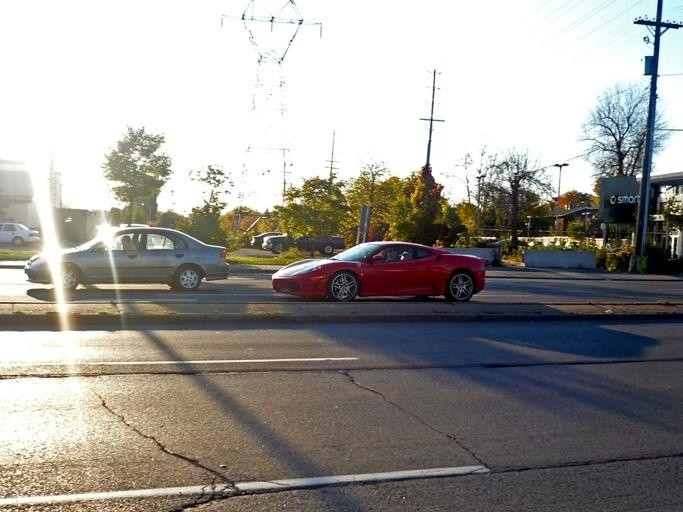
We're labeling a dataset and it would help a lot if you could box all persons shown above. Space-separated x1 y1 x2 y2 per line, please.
122 235 133 250
383 247 402 263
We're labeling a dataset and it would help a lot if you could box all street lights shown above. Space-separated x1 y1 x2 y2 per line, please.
476 175 485 224
552 162 569 234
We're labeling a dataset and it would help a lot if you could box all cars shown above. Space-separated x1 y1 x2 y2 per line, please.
252 231 345 254
25 227 229 292
273 241 489 301
0 223 40 246
